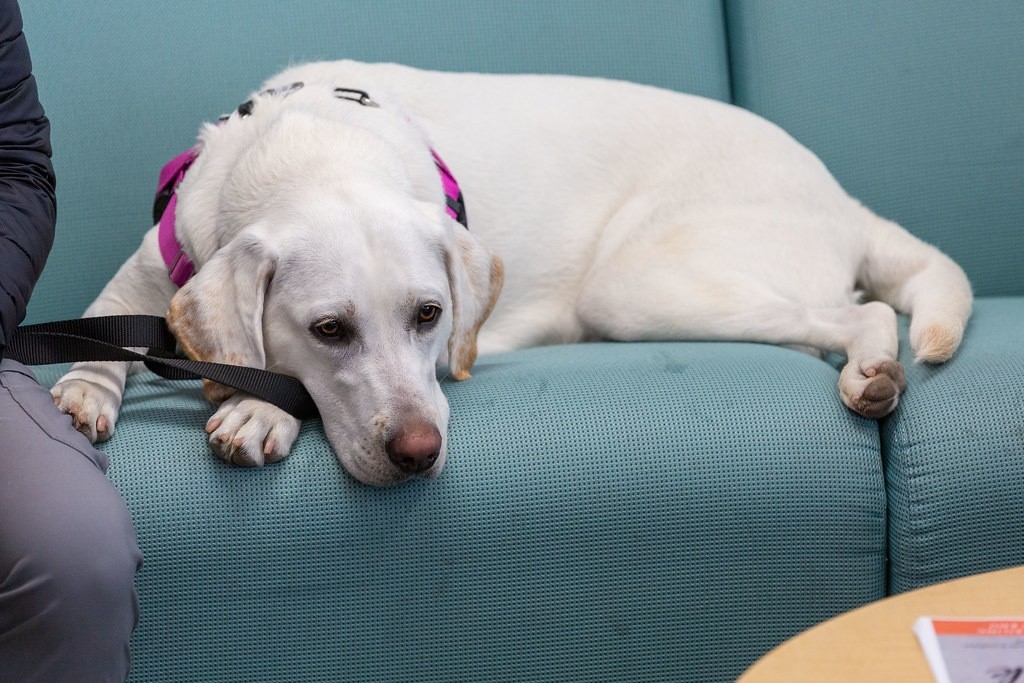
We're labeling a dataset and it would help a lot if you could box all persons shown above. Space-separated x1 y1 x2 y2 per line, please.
0 0 144 683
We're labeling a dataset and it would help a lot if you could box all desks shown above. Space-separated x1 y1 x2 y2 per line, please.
737 556 1023 683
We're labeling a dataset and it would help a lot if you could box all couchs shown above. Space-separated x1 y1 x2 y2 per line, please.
17 0 1024 683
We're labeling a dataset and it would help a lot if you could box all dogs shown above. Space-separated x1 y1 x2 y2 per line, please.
47 60 973 485
